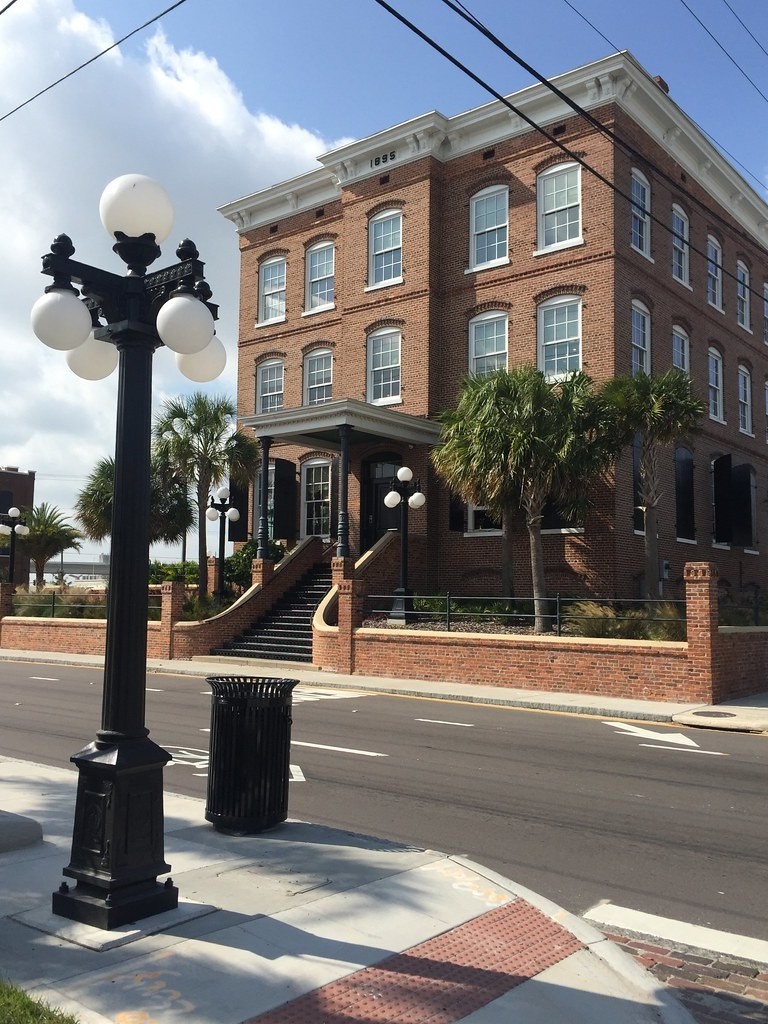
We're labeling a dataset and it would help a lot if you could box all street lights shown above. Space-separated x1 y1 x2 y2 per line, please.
206 486 240 604
384 467 426 623
32 173 227 931
0 506 29 584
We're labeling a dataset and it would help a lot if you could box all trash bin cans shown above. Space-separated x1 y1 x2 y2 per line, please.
202 675 300 836
